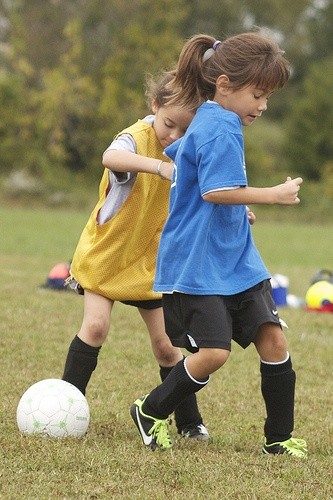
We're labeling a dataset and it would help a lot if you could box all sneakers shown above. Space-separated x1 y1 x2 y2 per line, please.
262 436 308 461
129 393 172 453
181 423 210 443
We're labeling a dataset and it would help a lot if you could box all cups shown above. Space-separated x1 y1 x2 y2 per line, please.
269 287 288 306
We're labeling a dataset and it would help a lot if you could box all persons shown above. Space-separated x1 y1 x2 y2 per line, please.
130 32 308 457
62 72 211 441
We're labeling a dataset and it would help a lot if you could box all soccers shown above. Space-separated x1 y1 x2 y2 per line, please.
305 281 333 310
15 378 91 439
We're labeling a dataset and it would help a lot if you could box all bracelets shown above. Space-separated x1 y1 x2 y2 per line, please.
157 160 164 175
